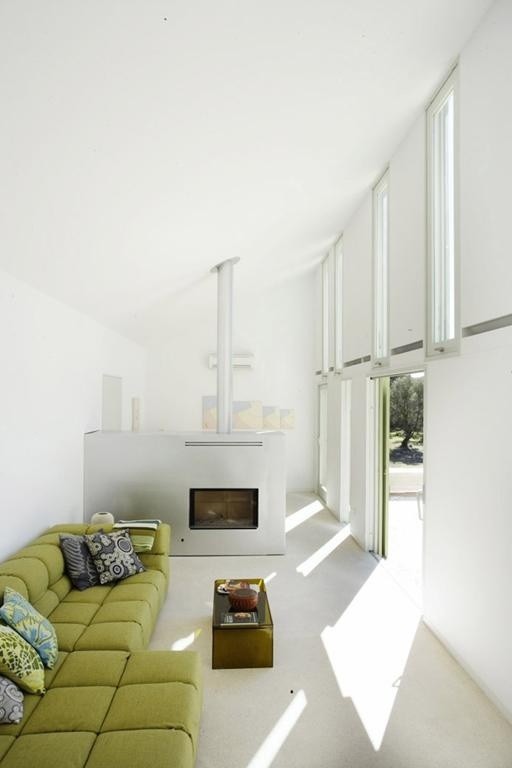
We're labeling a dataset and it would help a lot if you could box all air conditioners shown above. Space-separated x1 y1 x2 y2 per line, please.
208 352 254 369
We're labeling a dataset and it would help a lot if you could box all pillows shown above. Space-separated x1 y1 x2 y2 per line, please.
0 676 24 725
0 586 59 670
59 528 104 590
82 528 147 585
0 624 46 697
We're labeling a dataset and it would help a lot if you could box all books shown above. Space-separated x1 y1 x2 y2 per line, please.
219 611 259 627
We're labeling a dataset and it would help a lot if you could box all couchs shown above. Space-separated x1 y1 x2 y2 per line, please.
0 650 201 767
0 520 171 657
